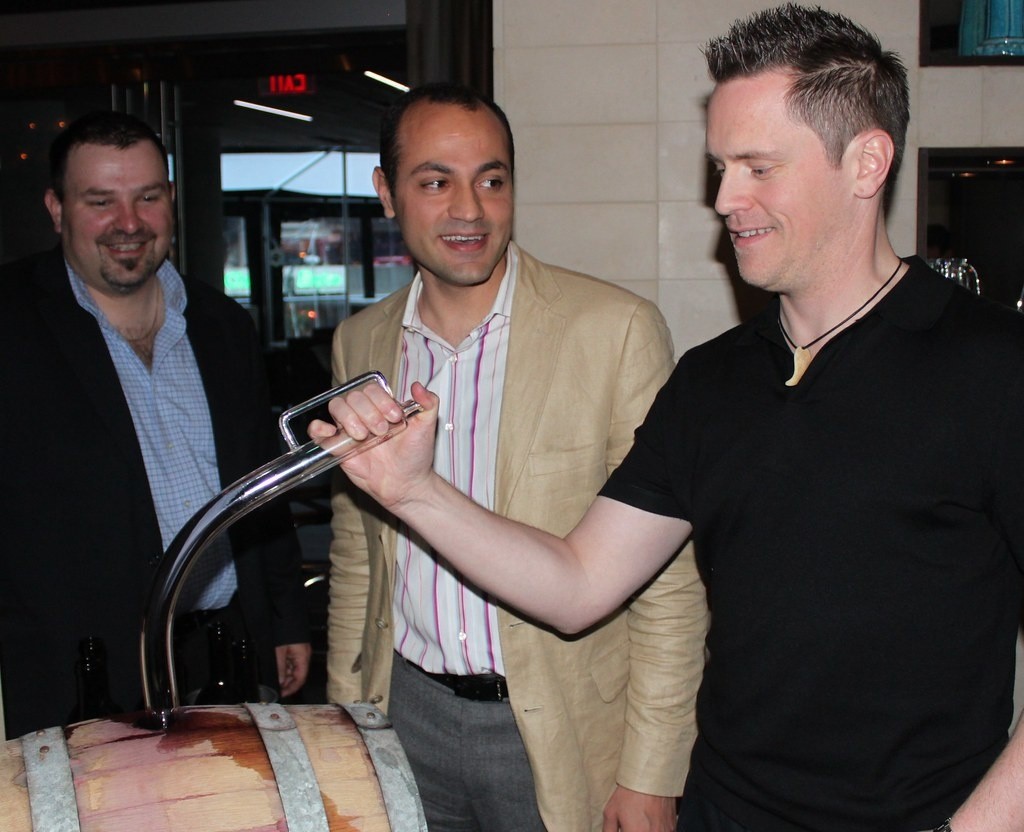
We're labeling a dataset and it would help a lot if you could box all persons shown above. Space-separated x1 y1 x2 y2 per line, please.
308 0 1024 832
0 108 314 740
323 79 714 832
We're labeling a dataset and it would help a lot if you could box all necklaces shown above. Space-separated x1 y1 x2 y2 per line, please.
778 256 903 387
